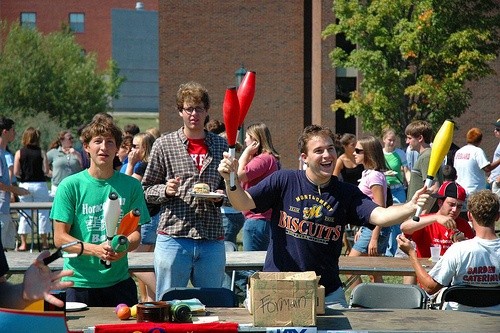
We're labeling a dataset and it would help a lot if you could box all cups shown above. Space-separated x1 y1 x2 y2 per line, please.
430 247 441 263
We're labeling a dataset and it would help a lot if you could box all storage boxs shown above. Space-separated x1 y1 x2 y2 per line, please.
249 271 319 328
247 285 325 314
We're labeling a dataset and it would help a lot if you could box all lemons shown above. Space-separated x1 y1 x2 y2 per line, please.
130 305 137 318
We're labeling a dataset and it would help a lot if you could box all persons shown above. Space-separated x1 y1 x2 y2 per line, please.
345 136 390 289
49 118 151 307
396 189 500 312
0 241 74 310
332 133 364 258
205 120 245 245
46 130 82 197
0 119 28 249
441 165 457 183
142 81 226 301
488 118 500 199
14 126 51 252
237 122 281 293
399 181 475 289
218 125 434 309
380 125 409 258
454 128 500 197
125 132 157 302
394 120 443 285
75 112 141 175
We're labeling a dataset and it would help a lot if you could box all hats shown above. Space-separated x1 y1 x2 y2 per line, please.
430 181 466 203
490 118 500 127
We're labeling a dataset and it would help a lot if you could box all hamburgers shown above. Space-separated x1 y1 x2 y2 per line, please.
194 182 210 194
450 231 464 243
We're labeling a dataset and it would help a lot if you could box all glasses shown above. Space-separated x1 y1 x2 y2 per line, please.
131 143 140 148
182 106 206 116
492 127 500 131
67 136 74 140
354 147 365 154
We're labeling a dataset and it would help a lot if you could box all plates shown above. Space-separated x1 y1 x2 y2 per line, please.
384 172 399 175
188 192 225 198
66 302 88 311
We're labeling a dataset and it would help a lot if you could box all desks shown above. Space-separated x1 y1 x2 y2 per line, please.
0 200 500 333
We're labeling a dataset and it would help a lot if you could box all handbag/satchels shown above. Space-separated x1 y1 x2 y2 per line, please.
402 183 408 200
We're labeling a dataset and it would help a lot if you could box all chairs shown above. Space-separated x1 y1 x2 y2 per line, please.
427 286 500 313
349 283 426 308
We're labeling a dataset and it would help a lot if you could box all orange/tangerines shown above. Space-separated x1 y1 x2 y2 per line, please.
117 306 131 320
